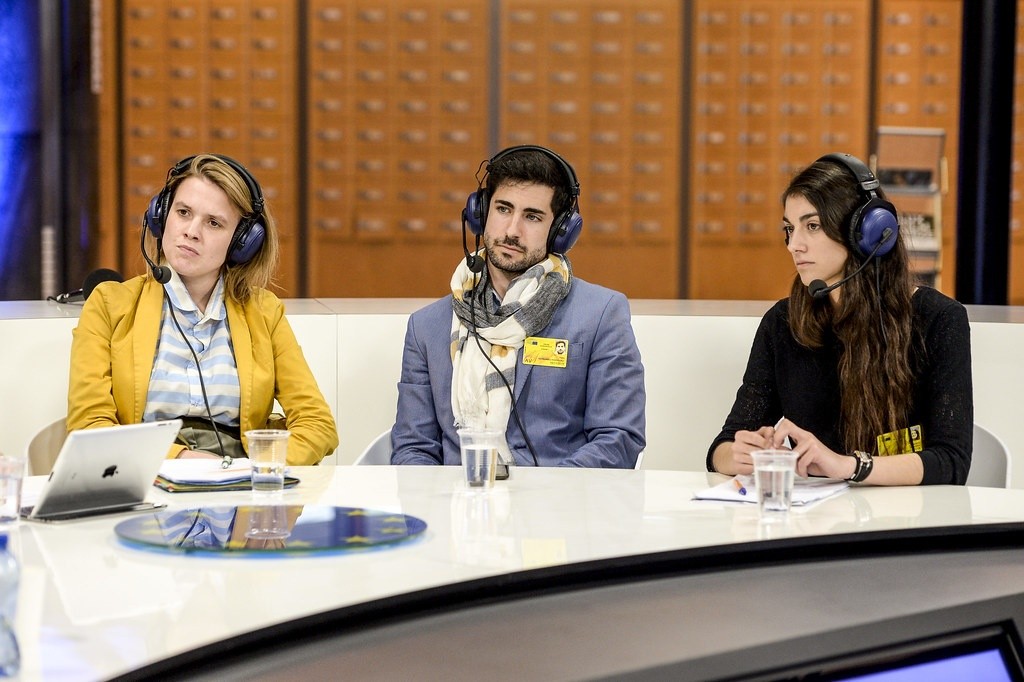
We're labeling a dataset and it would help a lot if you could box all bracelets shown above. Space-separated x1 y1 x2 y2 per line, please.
844 451 873 482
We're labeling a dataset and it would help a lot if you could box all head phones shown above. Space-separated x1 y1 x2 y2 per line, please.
784 151 901 260
466 145 583 254
147 153 267 267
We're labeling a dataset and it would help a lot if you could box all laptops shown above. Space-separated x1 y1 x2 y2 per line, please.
15 417 186 522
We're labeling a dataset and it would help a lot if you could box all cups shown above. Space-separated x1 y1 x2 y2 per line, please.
244 428 292 494
246 504 291 539
749 447 800 513
456 427 505 488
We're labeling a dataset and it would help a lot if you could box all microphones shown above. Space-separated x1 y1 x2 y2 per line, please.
808 228 893 298
141 212 172 284
57 268 124 304
462 208 485 273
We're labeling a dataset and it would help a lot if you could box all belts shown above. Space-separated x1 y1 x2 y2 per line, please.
174 415 242 440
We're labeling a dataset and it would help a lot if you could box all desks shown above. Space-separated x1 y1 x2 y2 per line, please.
0 464 1024 682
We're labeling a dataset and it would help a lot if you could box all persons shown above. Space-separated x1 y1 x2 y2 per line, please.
706 153 974 487
391 145 646 469
66 153 339 466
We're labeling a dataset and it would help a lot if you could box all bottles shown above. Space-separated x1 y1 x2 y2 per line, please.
0 533 19 678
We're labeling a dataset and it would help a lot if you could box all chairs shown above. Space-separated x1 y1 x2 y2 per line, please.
26 416 1004 490
